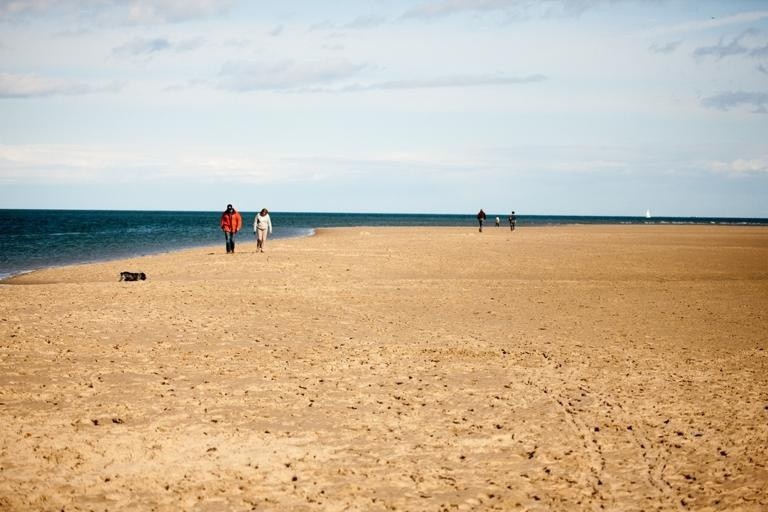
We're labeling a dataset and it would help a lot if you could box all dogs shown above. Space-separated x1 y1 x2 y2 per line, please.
118 271 147 282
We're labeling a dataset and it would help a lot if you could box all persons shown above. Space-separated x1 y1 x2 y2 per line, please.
478 209 486 232
253 209 272 252
495 215 499 228
221 205 242 254
509 211 516 231
